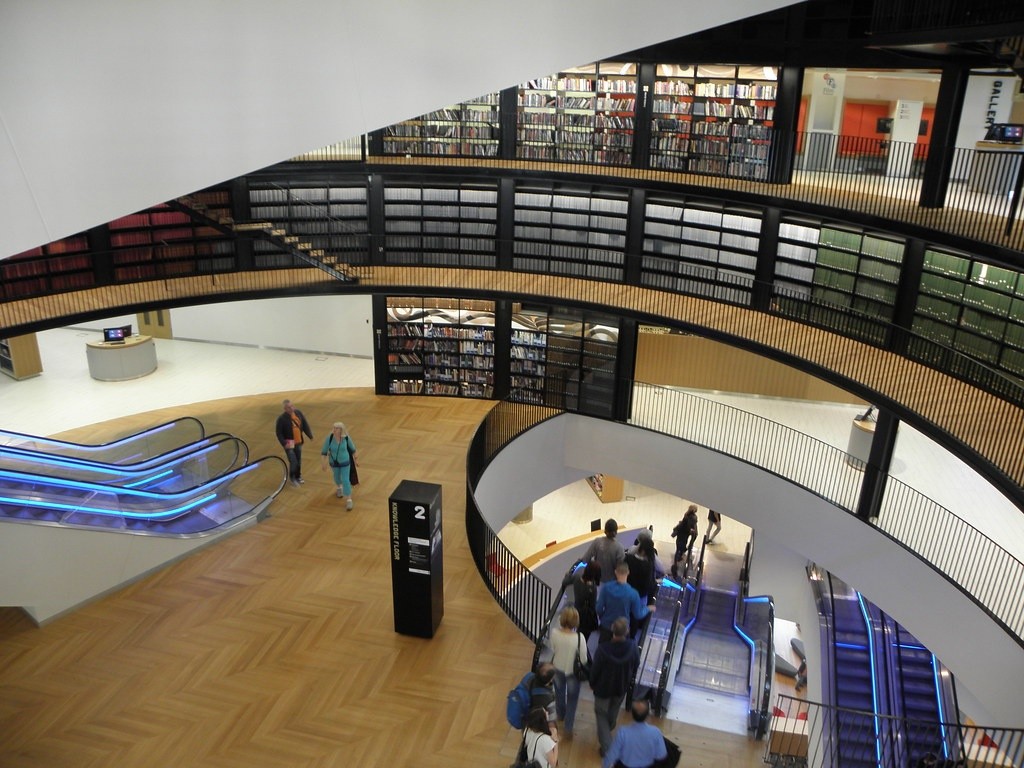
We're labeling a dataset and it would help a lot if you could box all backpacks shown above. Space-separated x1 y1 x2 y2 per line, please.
507 672 551 730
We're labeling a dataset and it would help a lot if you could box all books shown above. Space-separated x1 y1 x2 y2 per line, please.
249 188 367 267
108 192 237 283
0 231 94 298
386 322 619 420
772 223 905 340
383 187 497 267
642 204 763 305
513 192 628 282
907 250 1024 403
384 78 778 179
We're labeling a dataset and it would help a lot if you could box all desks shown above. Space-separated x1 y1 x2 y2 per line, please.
966 141 1024 195
848 417 901 473
85 335 157 381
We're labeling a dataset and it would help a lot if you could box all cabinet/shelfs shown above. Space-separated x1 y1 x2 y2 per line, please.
367 61 805 185
371 293 638 423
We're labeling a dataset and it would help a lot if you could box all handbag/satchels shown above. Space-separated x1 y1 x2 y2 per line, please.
516 728 528 764
574 632 592 681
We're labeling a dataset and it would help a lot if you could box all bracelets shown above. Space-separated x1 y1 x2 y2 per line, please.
554 739 559 743
355 457 358 459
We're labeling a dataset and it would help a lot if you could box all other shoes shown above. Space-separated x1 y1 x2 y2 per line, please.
296 478 304 483
346 499 352 510
337 489 343 497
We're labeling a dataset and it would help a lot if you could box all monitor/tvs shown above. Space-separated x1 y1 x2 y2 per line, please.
918 119 928 135
984 123 1000 140
999 123 1024 142
876 117 894 133
118 325 131 337
103 328 124 342
862 405 876 421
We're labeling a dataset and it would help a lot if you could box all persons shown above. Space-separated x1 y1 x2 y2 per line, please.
674 505 698 562
276 399 313 488
589 618 641 757
523 708 560 768
562 563 602 643
795 658 807 692
668 565 683 585
550 606 587 740
571 519 667 645
916 752 968 768
519 662 557 736
706 510 722 545
321 422 359 511
602 699 666 768
